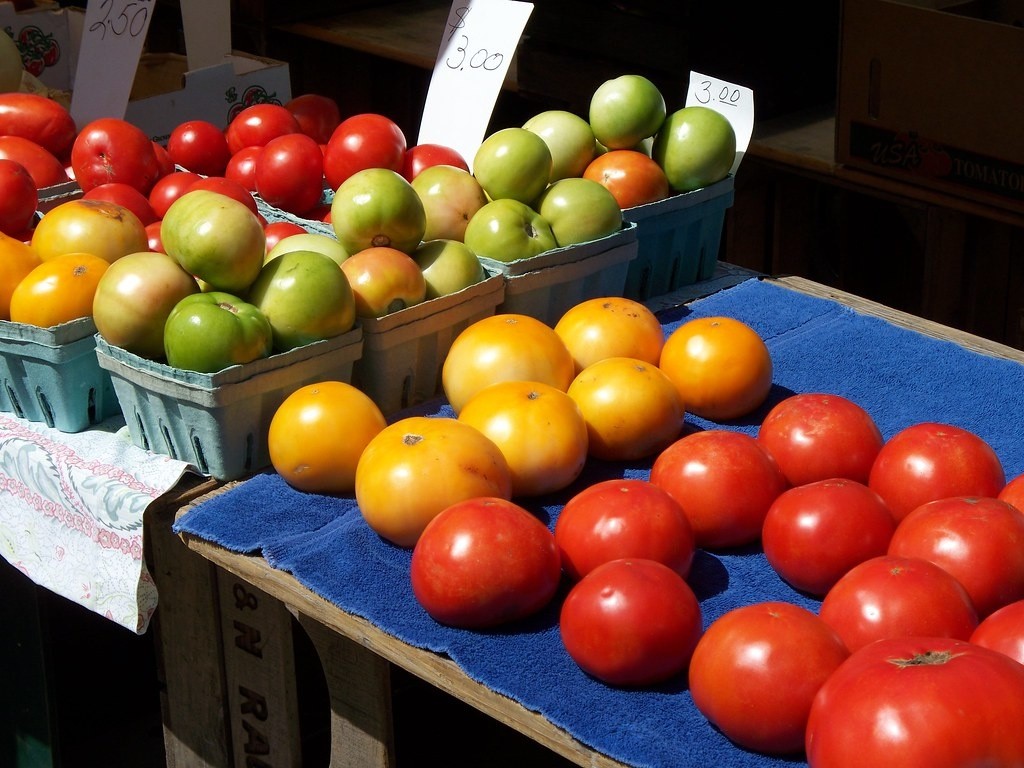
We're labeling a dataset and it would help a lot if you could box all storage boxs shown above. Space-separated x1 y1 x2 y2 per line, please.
0 0 292 149
0 165 736 480
836 0 1024 214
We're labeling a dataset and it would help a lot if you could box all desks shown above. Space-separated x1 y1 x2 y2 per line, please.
175 277 1024 768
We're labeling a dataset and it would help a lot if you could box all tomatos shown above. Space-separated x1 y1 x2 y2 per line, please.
1 76 1024 768
